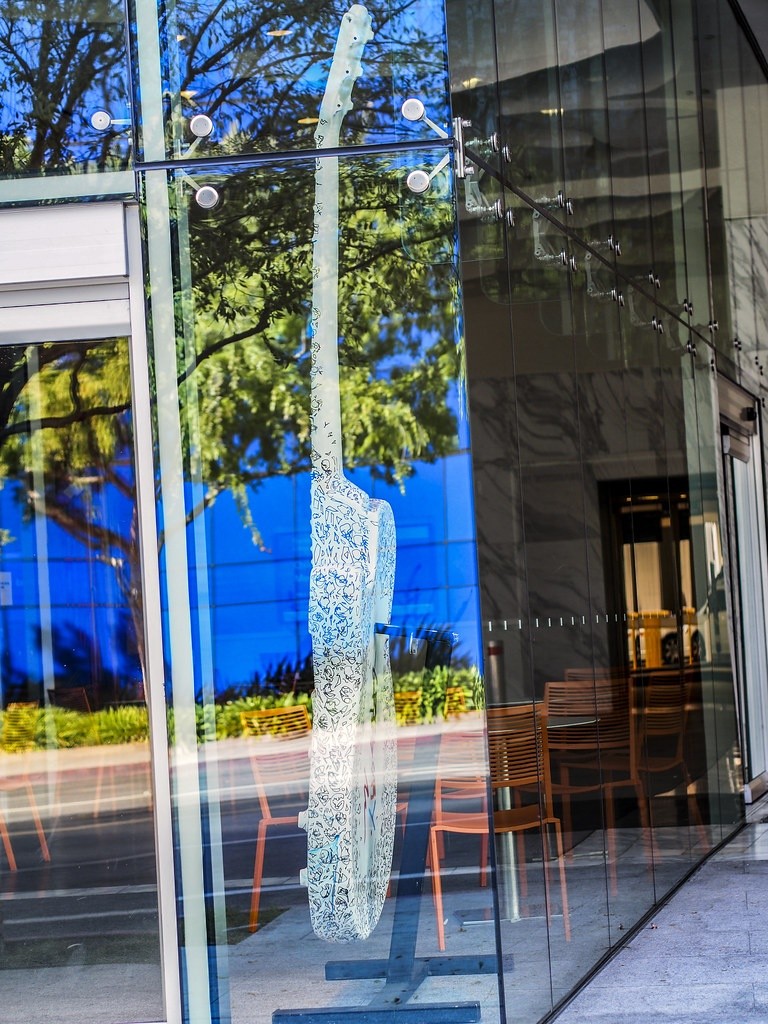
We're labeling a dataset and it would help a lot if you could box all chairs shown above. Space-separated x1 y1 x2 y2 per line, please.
0 659 734 957
